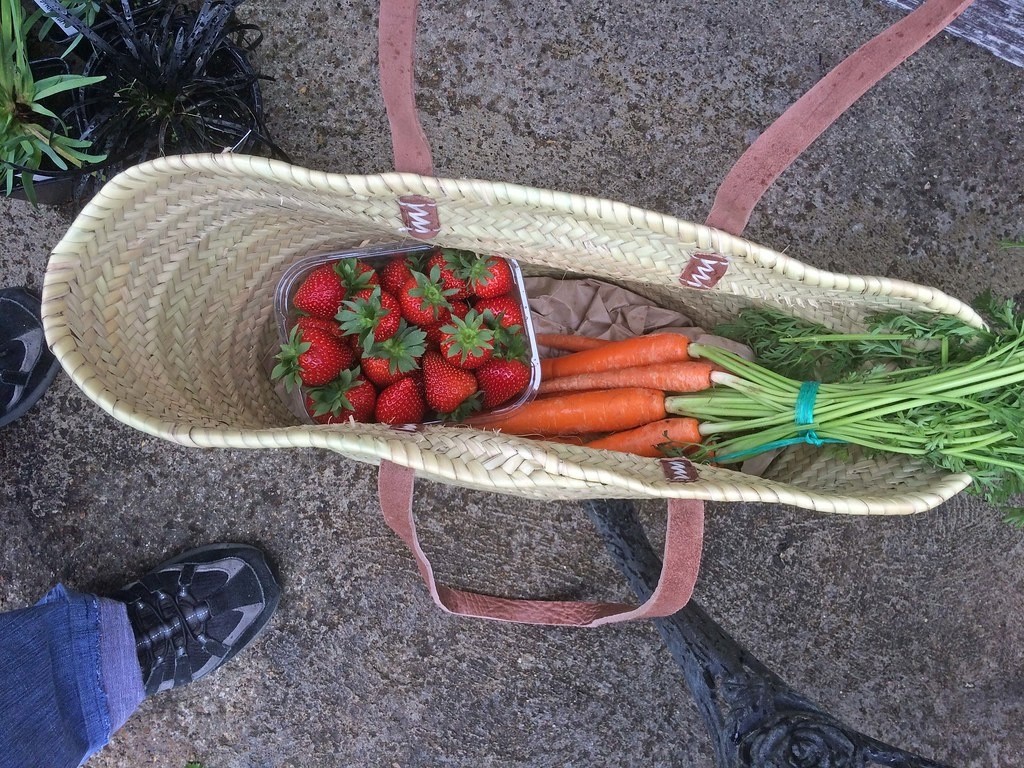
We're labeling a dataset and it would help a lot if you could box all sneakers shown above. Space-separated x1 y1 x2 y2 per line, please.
0 287 62 430
99 540 287 698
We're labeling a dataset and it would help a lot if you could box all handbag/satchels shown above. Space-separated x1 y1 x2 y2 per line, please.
41 0 995 627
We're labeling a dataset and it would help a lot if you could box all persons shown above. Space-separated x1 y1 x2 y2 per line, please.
0 287 283 768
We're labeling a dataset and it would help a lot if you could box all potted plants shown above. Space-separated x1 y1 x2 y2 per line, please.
0 1 293 211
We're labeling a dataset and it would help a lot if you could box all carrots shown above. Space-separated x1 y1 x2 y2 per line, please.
453 332 731 465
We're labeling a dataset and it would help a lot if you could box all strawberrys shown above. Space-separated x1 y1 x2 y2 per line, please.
271 247 530 425
